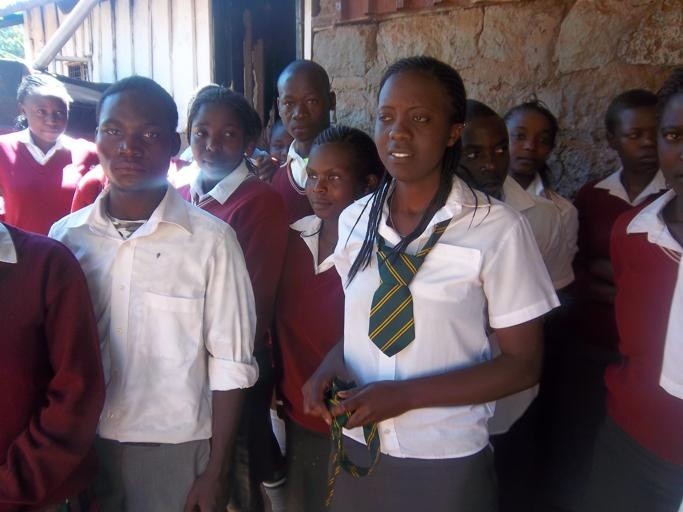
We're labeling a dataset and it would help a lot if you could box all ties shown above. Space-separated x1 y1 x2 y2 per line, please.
369 219 451 357
324 377 380 506
